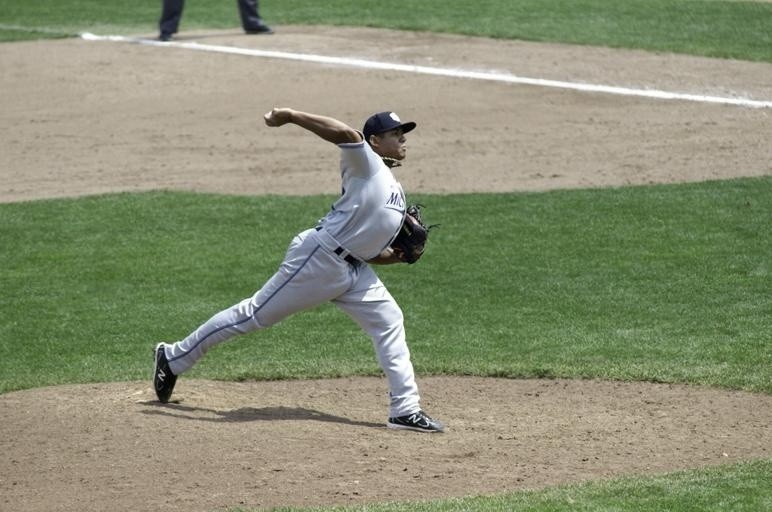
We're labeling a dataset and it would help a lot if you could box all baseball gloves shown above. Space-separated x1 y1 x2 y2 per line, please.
390 205 441 264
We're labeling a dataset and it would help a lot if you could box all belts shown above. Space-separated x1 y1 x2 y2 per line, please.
315 225 362 267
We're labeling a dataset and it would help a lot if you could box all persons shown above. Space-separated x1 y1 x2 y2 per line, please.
153 0 274 41
149 101 449 435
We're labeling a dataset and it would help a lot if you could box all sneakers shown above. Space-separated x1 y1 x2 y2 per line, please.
386 409 449 434
157 31 174 42
245 23 274 34
151 341 178 404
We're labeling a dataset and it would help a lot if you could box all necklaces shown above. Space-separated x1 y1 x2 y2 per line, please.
380 154 404 168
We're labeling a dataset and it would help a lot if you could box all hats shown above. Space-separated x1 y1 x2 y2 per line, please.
362 110 417 135
